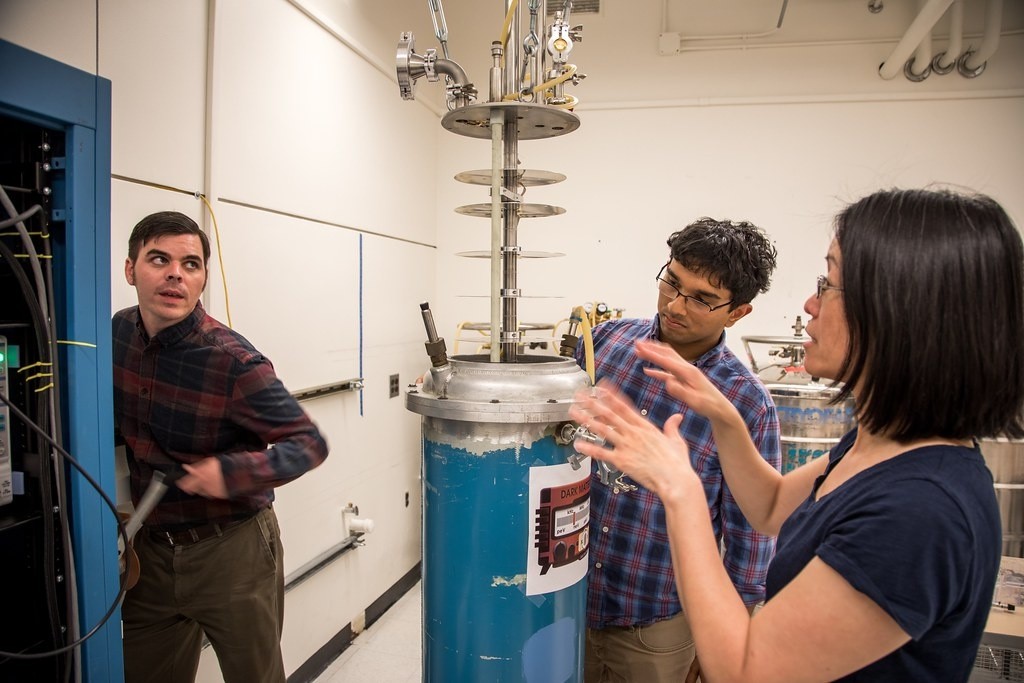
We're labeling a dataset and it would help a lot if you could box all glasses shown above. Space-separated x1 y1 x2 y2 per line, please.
656 262 735 314
815 274 846 300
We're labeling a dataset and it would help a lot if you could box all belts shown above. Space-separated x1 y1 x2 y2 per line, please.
143 516 238 548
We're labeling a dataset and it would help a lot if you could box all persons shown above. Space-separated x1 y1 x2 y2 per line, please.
573 216 782 683
568 181 1024 683
111 211 329 683
